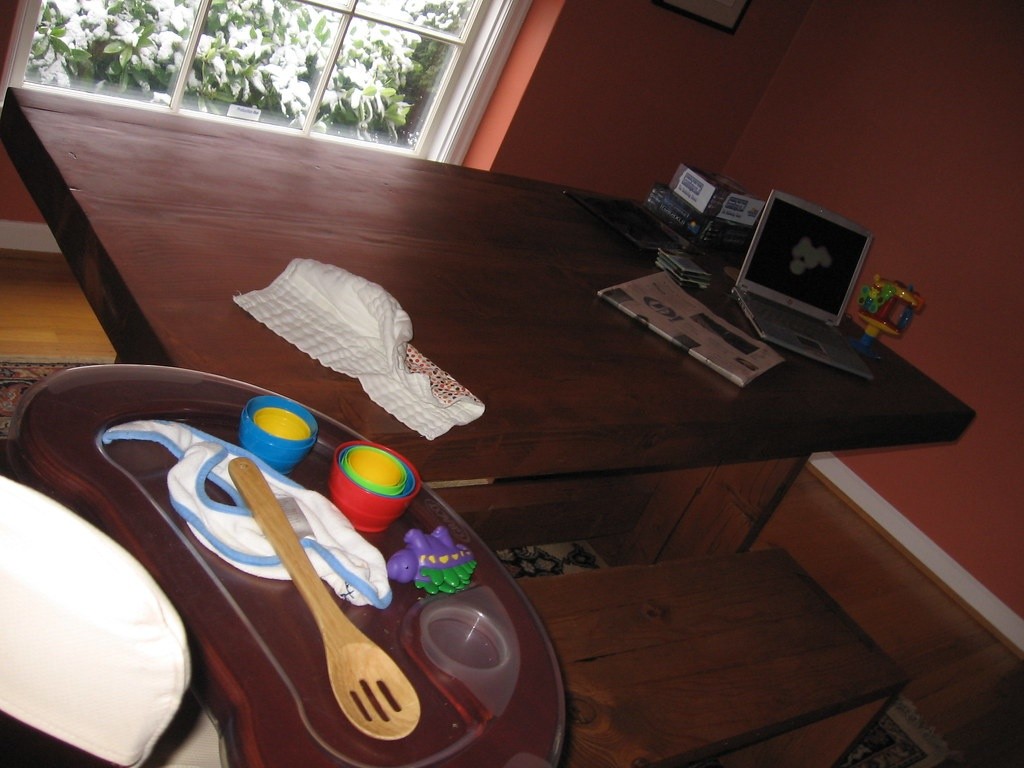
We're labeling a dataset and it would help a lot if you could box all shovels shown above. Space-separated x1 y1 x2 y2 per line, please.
228 457 423 740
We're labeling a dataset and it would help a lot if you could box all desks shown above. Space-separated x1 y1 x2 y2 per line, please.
0 84 980 565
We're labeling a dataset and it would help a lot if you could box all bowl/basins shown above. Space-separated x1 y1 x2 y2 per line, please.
328 441 421 532
238 395 319 473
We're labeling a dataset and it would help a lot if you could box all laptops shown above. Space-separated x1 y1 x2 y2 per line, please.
732 189 876 381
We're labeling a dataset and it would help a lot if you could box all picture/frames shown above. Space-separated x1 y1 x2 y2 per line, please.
649 0 751 35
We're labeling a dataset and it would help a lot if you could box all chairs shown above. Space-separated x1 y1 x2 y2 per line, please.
0 365 565 768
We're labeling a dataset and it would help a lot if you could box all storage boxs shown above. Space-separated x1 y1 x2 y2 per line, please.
641 183 760 252
669 163 765 229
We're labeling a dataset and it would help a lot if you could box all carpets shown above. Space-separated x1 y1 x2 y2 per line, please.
0 357 965 768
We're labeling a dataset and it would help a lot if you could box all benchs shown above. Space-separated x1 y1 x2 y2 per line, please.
509 538 913 767
